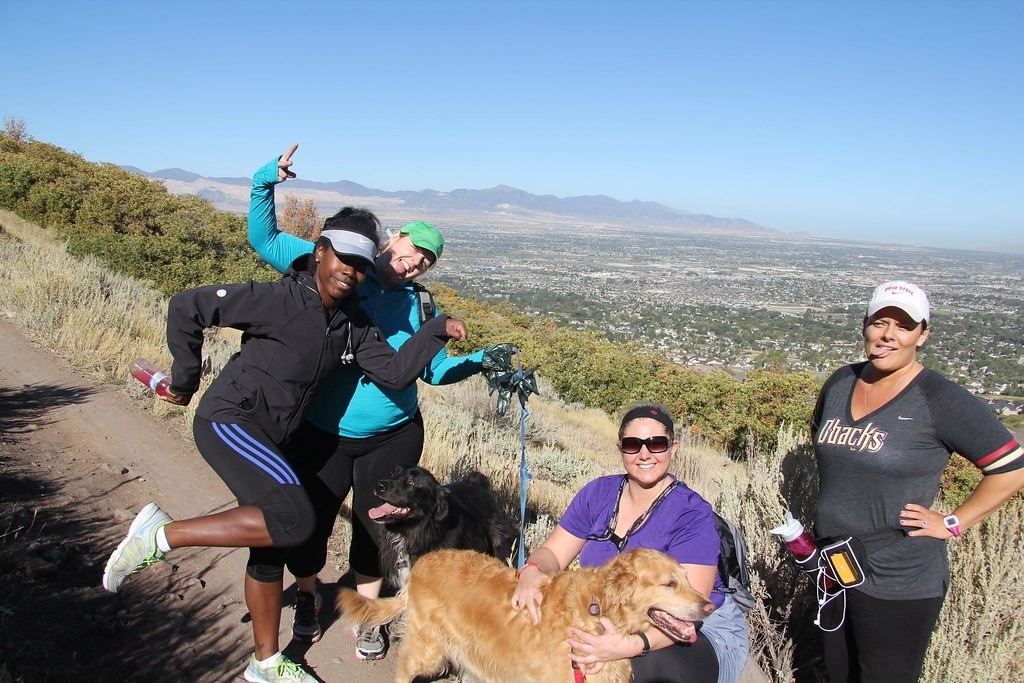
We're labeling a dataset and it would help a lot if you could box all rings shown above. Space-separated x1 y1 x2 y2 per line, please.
921 521 927 526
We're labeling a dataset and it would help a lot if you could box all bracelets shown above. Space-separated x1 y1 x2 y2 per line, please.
632 631 650 657
516 562 542 580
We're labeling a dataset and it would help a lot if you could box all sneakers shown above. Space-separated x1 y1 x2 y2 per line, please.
244 652 320 683
103 501 175 594
352 621 386 659
293 585 322 642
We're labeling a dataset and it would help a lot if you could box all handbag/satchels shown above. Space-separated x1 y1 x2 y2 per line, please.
805 520 923 587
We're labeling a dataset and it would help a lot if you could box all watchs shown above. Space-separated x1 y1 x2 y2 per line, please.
943 513 960 537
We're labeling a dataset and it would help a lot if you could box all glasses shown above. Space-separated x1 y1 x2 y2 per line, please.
618 436 674 454
335 253 369 274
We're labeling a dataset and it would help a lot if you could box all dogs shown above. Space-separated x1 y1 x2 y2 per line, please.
334 548 715 683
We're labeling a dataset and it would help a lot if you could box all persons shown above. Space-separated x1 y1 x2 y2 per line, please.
248 144 517 659
811 280 1024 683
104 208 468 683
511 405 749 683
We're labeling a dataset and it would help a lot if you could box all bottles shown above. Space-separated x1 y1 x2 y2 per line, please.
768 511 836 590
129 358 174 396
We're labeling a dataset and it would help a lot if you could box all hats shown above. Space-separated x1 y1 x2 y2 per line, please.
869 281 930 325
320 226 378 265
400 221 444 270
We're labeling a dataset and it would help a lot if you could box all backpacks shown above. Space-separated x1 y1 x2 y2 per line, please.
712 511 755 612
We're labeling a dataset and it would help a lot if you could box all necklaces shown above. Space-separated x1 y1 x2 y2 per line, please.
865 370 902 414
